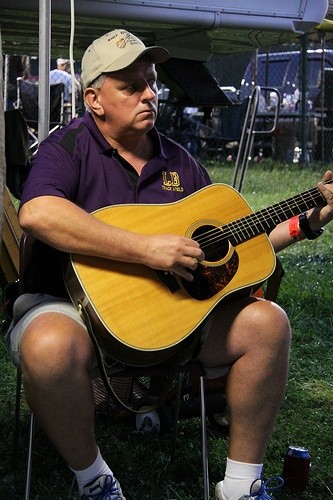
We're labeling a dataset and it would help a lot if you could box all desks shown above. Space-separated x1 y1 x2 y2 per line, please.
254 113 327 158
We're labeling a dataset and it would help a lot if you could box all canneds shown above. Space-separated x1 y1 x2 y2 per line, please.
281 446 312 490
135 405 181 438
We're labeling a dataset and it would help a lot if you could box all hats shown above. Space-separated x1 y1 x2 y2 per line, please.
56 58 75 64
80 28 170 89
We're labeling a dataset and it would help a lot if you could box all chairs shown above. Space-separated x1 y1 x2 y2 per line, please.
187 103 250 165
14 231 265 500
13 77 72 156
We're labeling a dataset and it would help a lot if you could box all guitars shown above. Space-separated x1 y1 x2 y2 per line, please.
62 179 332 368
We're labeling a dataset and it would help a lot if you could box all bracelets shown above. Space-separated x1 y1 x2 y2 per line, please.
289 215 305 241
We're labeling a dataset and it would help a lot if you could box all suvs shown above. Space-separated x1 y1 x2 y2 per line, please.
215 49 332 115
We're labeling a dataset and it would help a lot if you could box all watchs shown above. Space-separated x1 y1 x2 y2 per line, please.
300 212 325 240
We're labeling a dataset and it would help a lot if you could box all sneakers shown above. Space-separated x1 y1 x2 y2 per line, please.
213 477 284 500
80 474 126 500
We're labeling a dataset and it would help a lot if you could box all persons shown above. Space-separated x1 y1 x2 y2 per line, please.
8 56 82 120
6 29 333 500
